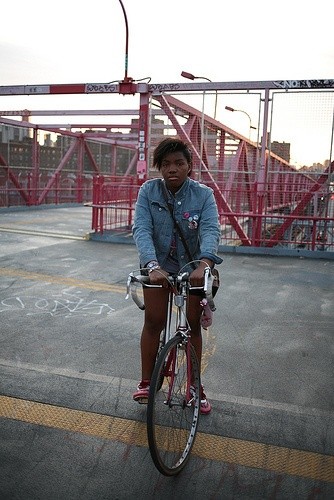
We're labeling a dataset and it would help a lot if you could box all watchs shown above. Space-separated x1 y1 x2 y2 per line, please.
145 261 158 269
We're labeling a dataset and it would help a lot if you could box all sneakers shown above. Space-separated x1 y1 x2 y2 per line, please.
188 387 212 415
132 378 151 401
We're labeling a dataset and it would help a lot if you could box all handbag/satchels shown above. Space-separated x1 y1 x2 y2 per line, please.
205 267 219 299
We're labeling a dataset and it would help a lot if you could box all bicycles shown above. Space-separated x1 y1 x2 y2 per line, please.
123 258 219 477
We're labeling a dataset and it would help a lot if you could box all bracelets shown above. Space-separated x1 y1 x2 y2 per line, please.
148 266 161 274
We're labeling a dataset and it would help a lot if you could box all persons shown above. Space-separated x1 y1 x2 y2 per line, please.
134 138 224 414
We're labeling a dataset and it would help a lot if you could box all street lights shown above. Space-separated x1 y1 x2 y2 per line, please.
181 70 218 120
223 106 251 148
249 125 258 148
